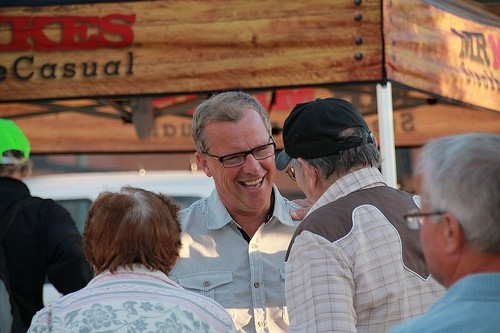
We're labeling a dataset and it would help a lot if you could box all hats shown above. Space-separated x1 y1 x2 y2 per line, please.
0 118 31 165
275 97 376 172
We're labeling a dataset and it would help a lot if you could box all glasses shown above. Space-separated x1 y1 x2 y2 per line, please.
202 136 277 168
403 211 446 232
285 166 295 182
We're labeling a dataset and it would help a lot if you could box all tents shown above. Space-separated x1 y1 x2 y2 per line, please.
0 1 500 193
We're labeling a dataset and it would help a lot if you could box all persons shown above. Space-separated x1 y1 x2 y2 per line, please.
275 97 449 333
27 186 238 333
386 132 499 333
0 119 94 333
165 91 308 333
